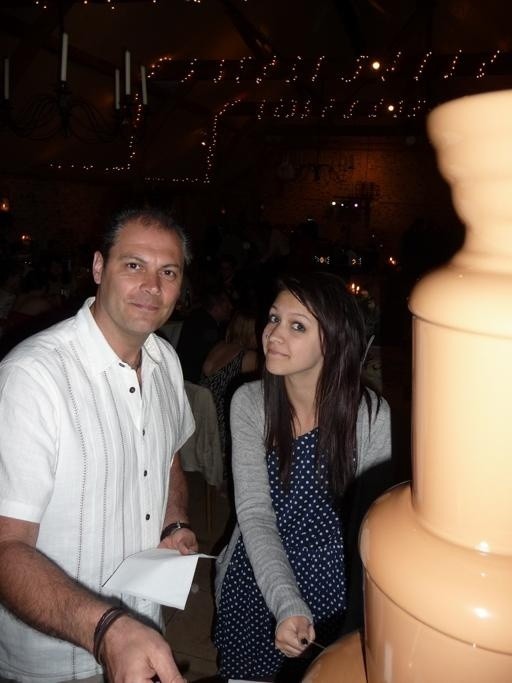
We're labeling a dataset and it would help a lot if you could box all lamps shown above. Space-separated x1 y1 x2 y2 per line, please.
0 0 148 145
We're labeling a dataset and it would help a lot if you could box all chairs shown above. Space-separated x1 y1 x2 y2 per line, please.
179 381 230 537
159 321 183 350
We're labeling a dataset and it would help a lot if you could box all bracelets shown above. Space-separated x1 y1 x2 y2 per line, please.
92 607 126 667
161 521 190 541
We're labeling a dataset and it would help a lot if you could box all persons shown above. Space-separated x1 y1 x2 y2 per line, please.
1 196 432 509
0 204 200 683
210 271 392 682
